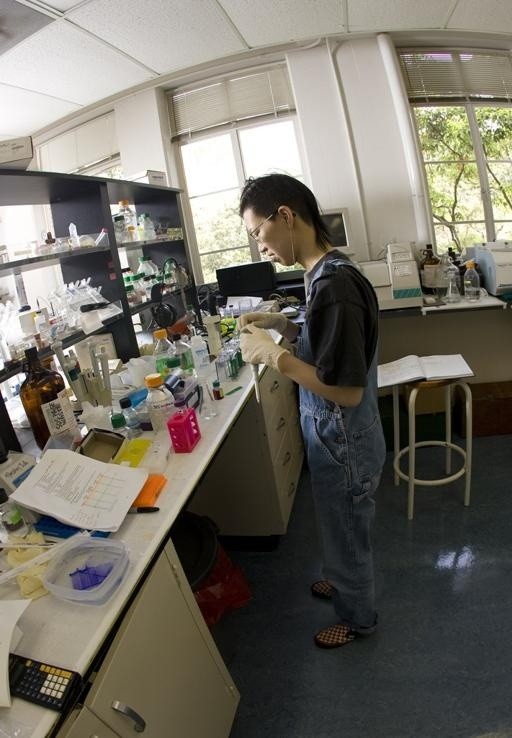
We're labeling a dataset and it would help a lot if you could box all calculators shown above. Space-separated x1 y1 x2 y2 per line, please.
8 652 82 713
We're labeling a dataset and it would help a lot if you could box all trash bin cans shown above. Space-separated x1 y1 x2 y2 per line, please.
172 510 252 626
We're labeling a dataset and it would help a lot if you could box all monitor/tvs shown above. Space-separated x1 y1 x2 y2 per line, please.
318 207 355 254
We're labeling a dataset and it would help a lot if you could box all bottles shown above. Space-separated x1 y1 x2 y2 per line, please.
67 223 81 249
19 347 82 455
145 329 247 432
118 200 157 240
222 304 236 332
0 246 9 265
0 488 29 539
121 255 188 302
94 227 107 248
420 243 485 305
44 231 57 254
111 397 144 439
185 305 198 325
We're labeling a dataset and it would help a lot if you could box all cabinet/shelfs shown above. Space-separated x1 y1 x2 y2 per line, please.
0 165 302 738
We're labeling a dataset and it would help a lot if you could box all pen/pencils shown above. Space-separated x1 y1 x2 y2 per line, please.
128 506 160 513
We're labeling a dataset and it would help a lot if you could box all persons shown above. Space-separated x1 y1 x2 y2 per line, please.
234 173 387 649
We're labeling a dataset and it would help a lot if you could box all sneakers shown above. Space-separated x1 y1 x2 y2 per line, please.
314 622 357 648
311 580 335 598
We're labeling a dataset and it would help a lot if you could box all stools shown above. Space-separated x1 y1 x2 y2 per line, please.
391 377 473 522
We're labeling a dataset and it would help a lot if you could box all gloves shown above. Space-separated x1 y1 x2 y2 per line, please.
236 311 288 371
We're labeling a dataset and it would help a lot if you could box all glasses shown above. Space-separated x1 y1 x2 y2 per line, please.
246 212 279 239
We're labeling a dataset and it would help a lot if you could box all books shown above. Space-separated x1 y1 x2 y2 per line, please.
377 353 474 389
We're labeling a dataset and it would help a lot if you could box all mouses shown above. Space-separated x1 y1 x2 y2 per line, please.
423 297 436 304
